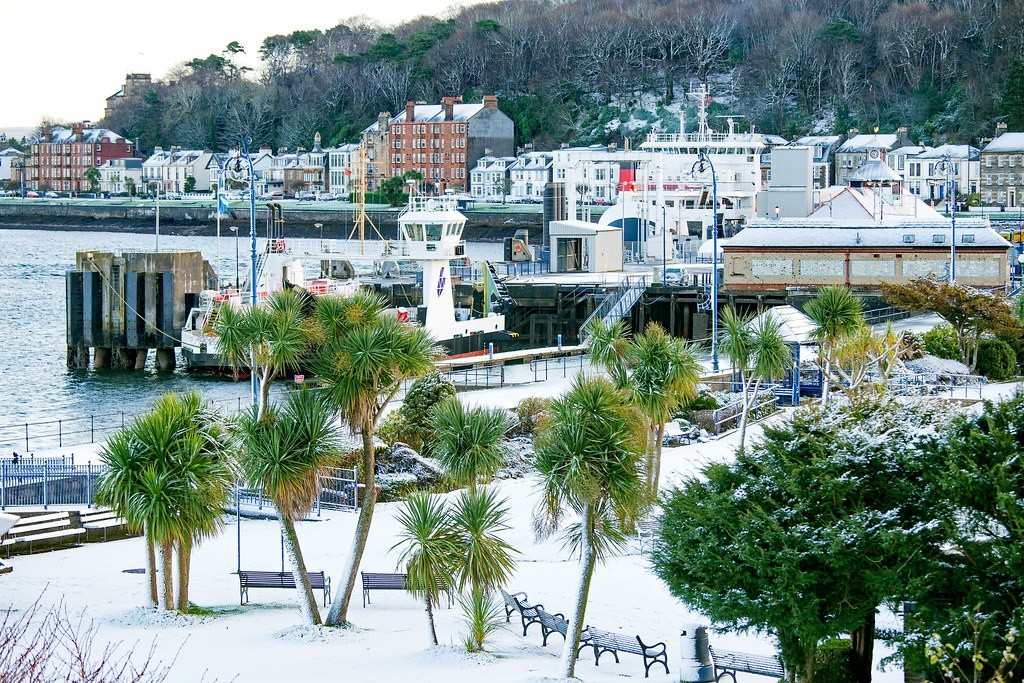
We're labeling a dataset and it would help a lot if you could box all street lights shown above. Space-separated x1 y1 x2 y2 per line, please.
230 226 239 293
932 152 956 287
315 223 323 254
690 149 721 375
232 135 258 425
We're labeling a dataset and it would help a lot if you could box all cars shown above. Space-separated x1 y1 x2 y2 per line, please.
45 192 59 198
0 187 43 198
228 190 349 202
168 193 181 199
486 196 540 203
584 199 615 206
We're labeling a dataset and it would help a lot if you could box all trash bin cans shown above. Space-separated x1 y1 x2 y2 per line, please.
678 623 715 683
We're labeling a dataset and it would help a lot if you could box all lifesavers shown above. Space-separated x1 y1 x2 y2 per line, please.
276 241 286 251
514 245 522 251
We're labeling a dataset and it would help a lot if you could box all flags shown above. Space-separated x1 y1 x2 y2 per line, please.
219 175 228 217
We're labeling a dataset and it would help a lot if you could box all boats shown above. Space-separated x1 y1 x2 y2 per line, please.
180 171 516 377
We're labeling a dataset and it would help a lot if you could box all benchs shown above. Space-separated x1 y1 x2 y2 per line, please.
237 570 332 608
535 605 593 658
708 645 785 683
498 585 541 636
655 422 692 447
633 521 655 555
360 570 454 609
8 512 87 554
79 503 128 542
588 626 670 678
